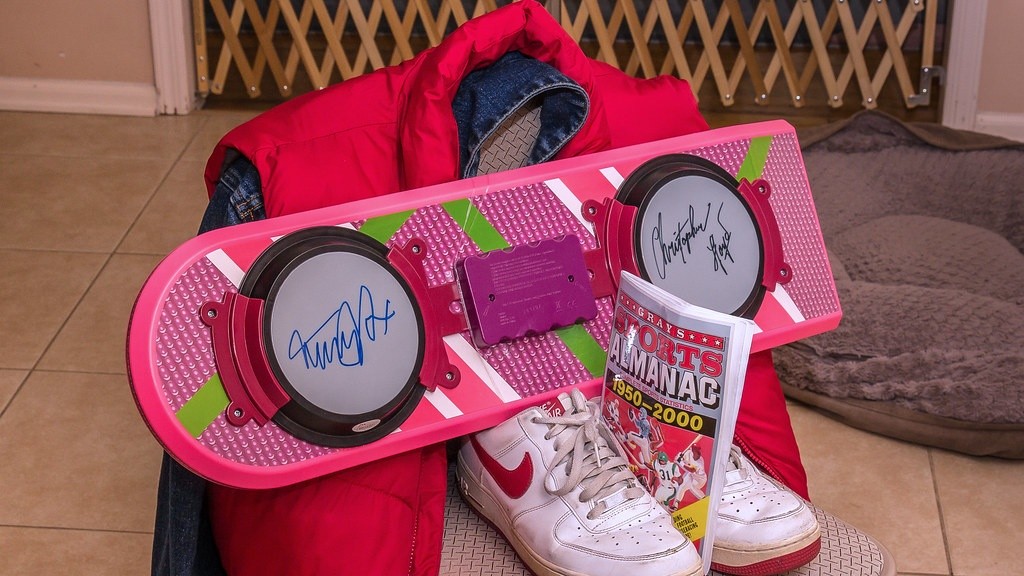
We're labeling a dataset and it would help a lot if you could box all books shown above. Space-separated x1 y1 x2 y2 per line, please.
595 269 764 576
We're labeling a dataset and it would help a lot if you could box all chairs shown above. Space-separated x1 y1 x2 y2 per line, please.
151 0 896 576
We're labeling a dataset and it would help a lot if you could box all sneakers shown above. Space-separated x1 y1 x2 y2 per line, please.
454 388 703 575
708 442 819 575
